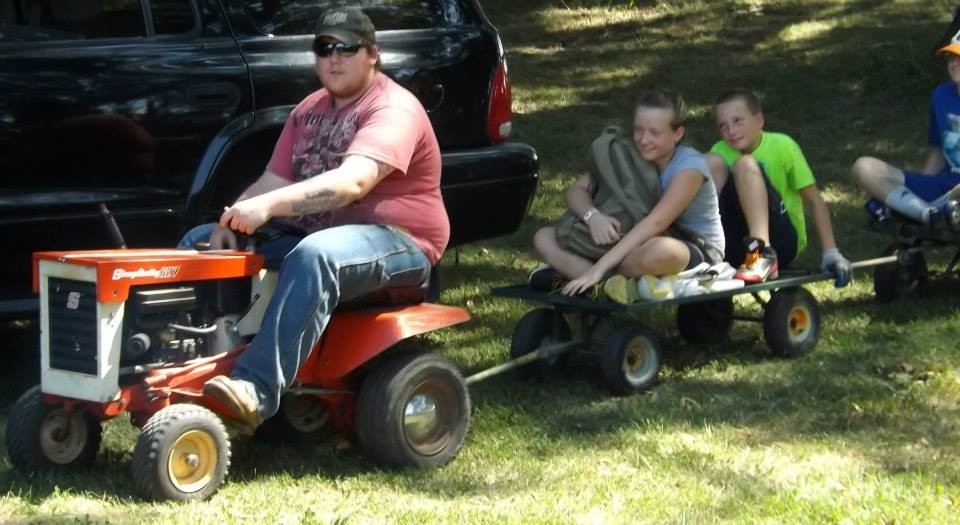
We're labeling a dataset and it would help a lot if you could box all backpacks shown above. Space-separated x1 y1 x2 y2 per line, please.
554 124 660 261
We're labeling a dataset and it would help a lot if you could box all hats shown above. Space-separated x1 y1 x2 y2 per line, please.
315 7 374 46
936 30 960 56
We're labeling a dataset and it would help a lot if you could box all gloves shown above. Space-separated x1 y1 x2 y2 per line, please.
821 248 853 288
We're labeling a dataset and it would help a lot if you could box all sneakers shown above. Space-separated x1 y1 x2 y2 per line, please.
735 234 779 283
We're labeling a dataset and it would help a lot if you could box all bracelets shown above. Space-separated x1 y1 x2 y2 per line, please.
583 208 599 224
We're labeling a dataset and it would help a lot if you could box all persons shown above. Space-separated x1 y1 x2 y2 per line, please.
852 30 960 230
534 89 726 304
705 85 853 289
177 6 451 429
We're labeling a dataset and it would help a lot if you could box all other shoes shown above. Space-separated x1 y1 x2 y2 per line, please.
587 272 639 304
203 376 260 430
929 198 960 231
529 265 568 297
865 198 903 223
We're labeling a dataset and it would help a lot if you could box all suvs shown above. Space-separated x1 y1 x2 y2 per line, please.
0 0 543 321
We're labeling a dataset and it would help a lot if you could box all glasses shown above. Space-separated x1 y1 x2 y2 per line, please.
943 54 955 62
313 41 360 57
